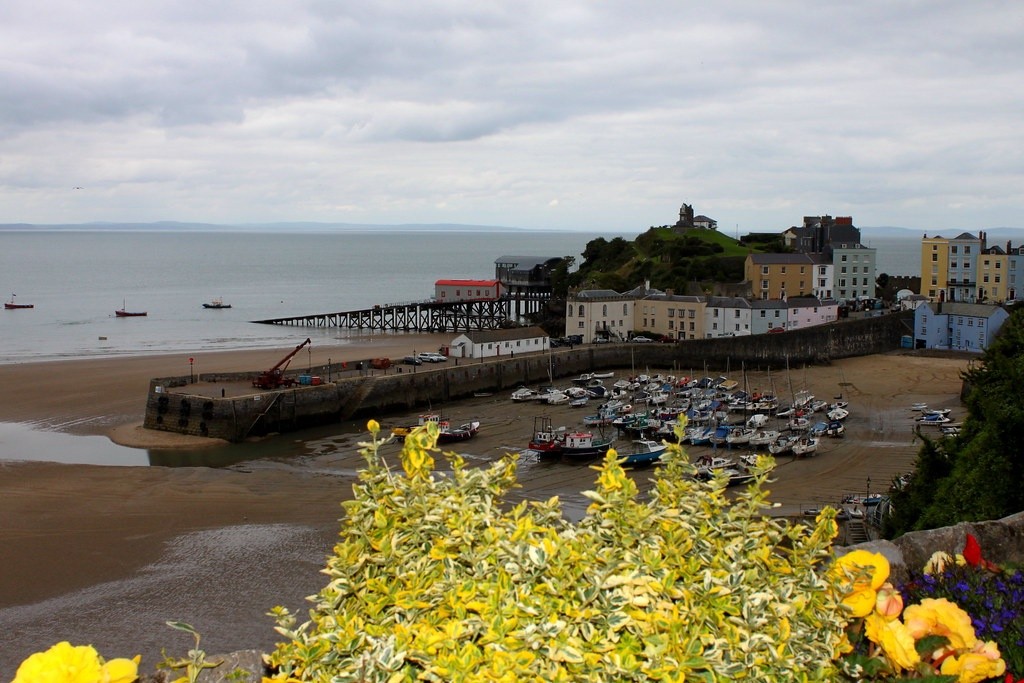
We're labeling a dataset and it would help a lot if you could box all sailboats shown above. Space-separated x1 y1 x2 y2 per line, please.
547 342 569 405
622 346 850 456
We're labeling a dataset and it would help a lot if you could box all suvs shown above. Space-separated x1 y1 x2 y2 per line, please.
419 351 439 363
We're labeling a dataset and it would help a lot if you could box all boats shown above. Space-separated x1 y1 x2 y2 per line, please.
938 423 964 440
912 402 927 406
912 406 928 410
563 372 640 427
691 456 733 479
799 493 887 519
202 296 232 308
528 416 615 464
707 453 773 486
510 388 549 401
917 415 956 426
919 408 951 417
115 300 147 316
393 414 480 442
4 292 34 309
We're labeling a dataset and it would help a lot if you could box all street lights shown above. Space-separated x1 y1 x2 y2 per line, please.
328 358 331 382
866 476 871 511
413 350 416 373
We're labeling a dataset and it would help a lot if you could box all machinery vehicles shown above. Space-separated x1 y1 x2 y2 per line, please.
252 338 311 388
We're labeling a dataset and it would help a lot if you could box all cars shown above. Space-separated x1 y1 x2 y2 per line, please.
592 337 608 344
633 336 653 343
404 356 422 365
434 353 448 362
